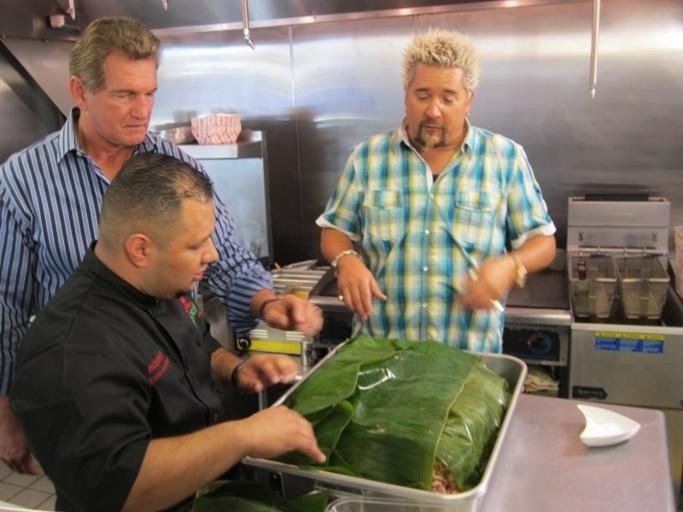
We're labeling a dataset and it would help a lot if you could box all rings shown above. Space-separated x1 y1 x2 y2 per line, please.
338 296 344 301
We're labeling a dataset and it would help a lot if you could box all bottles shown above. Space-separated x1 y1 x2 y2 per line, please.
571 259 592 321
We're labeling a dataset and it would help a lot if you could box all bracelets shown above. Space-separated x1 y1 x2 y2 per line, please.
229 359 247 385
328 250 362 276
258 295 281 314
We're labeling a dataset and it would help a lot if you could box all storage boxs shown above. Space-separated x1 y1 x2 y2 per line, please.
236 336 528 512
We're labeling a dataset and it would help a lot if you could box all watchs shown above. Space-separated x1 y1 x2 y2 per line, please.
510 250 528 288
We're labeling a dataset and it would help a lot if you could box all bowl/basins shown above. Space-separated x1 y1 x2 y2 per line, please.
154 120 194 146
190 113 239 146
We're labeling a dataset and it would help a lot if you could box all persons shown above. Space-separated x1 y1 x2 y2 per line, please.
7 151 326 511
0 16 325 475
315 27 556 353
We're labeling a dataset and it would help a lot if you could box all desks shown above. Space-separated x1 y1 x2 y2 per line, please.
470 391 676 512
